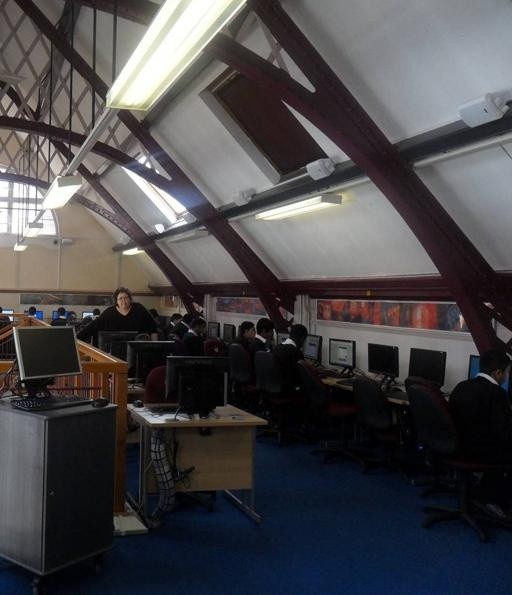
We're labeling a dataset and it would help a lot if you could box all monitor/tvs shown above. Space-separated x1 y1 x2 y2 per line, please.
409 348 446 386
300 335 326 369
1 309 94 321
468 355 512 393
127 340 175 386
13 326 83 401
329 339 356 375
160 314 298 343
368 343 399 387
99 331 138 361
166 356 232 416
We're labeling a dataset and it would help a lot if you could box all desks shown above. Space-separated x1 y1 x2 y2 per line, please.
128 383 146 394
322 375 411 406
127 404 268 530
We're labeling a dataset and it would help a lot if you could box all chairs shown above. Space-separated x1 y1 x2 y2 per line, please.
206 336 286 445
423 404 511 542
347 376 458 487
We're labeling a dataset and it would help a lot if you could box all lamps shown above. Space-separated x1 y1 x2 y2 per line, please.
38 4 92 213
103 1 249 113
20 102 46 240
10 149 30 254
165 224 210 244
256 188 347 225
121 246 145 257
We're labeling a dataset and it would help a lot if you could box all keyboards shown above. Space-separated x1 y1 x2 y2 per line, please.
383 389 409 400
337 378 356 386
150 405 182 414
11 396 94 411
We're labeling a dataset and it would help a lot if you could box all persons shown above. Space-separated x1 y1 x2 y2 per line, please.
446 347 511 474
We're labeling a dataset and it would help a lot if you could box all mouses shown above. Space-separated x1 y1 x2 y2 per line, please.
93 398 108 408
133 400 144 407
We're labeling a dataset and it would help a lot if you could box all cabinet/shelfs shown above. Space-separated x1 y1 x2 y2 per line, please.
0 391 120 594
